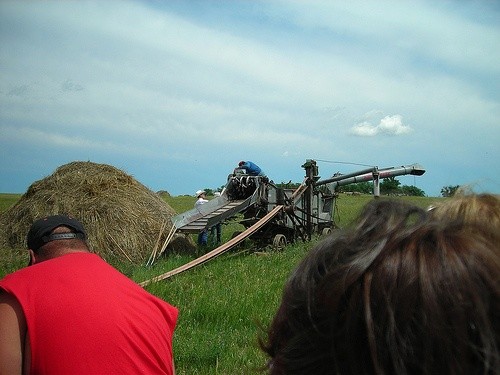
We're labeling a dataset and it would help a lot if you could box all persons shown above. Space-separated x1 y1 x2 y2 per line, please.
236 161 267 199
211 191 223 245
194 190 209 247
0 215 179 375
256 177 500 375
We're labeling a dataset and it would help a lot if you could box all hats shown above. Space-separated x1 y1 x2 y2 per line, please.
195 189 205 198
27 215 87 252
